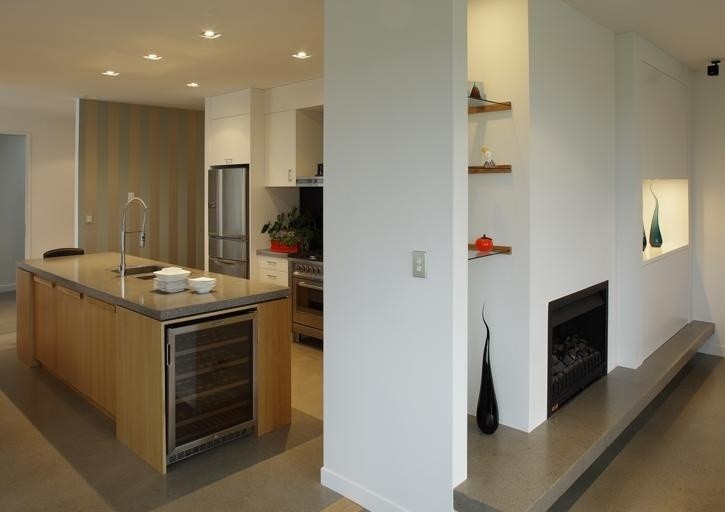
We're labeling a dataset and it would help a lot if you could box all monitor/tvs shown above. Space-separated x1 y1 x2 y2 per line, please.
299 188 323 251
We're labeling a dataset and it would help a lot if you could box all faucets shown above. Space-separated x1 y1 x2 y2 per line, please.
119 193 147 276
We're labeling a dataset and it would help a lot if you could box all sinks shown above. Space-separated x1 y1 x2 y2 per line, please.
135 274 157 281
109 263 161 276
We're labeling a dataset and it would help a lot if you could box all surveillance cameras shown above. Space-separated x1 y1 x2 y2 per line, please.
707 60 720 76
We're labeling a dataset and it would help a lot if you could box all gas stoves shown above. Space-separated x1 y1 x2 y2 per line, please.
288 251 322 275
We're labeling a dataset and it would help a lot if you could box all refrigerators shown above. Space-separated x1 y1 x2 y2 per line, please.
208 167 249 280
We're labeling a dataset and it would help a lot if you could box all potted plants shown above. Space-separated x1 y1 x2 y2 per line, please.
258 205 320 256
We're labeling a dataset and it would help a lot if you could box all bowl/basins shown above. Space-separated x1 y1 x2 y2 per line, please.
190 277 217 295
151 267 191 294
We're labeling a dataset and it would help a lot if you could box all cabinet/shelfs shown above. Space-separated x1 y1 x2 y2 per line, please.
254 253 288 284
266 76 326 188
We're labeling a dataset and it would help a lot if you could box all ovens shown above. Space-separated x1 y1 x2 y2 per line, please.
292 276 323 331
